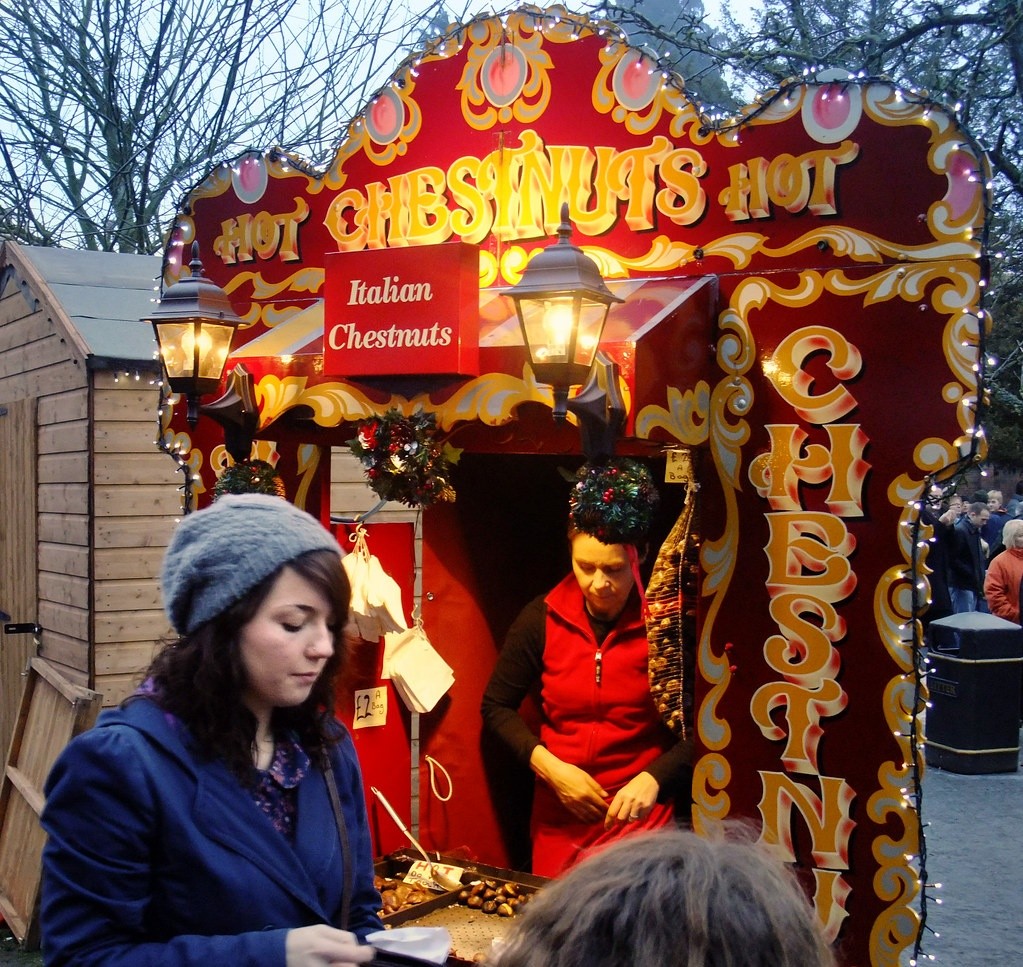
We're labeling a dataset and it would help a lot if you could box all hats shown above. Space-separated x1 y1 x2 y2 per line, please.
160 494 343 636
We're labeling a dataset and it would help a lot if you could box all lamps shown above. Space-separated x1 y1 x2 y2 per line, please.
138 241 241 425
503 204 623 425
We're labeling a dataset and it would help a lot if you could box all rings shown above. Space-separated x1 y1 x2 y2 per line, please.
629 814 639 819
581 811 589 817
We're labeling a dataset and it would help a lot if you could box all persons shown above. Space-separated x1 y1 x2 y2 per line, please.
917 482 1023 621
491 809 837 967
480 518 689 886
30 495 388 967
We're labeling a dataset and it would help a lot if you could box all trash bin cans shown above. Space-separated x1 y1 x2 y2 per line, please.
925 611 1023 777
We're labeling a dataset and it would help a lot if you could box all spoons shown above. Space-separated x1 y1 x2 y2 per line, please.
372 787 464 891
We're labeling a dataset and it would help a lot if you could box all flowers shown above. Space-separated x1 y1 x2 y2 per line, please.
357 412 457 519
210 455 286 506
567 453 659 545
